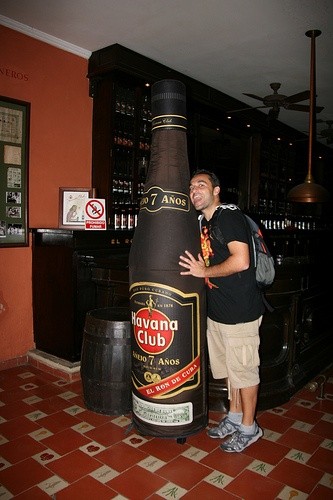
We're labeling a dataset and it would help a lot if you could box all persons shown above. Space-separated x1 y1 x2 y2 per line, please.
179 169 267 453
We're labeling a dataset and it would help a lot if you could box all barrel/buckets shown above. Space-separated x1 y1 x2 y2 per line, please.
81 306 131 415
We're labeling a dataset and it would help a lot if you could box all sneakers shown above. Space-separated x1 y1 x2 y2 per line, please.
207 416 241 438
219 424 263 452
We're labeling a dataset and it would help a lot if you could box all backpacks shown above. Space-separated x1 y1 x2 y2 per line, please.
198 203 277 291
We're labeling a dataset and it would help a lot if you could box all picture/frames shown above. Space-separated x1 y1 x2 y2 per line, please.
58 187 93 230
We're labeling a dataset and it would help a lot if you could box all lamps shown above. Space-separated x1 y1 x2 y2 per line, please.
287 30 330 202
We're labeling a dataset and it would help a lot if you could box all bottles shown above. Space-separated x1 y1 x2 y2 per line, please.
130 78 211 436
258 199 317 230
109 92 151 231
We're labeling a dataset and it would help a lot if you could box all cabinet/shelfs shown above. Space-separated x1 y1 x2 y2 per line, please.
88 45 333 233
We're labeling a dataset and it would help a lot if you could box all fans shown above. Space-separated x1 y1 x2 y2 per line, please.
226 83 325 120
